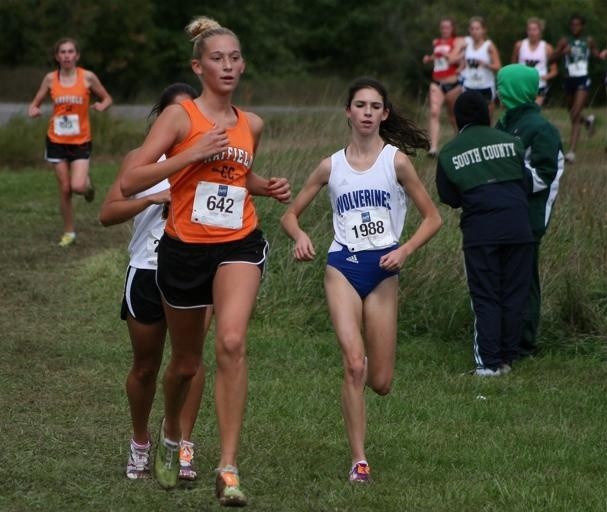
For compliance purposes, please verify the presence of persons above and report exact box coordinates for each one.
[27,38,113,250]
[119,15,292,508]
[435,89,542,376]
[419,14,607,163]
[281,78,443,488]
[492,63,565,356]
[96,81,215,482]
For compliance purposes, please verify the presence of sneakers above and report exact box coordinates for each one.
[216,465,247,505]
[473,368,501,376]
[179,440,197,481]
[127,432,151,479]
[586,114,595,138]
[59,232,76,248]
[86,173,94,202]
[563,151,576,162]
[155,416,179,488]
[349,461,369,484]
